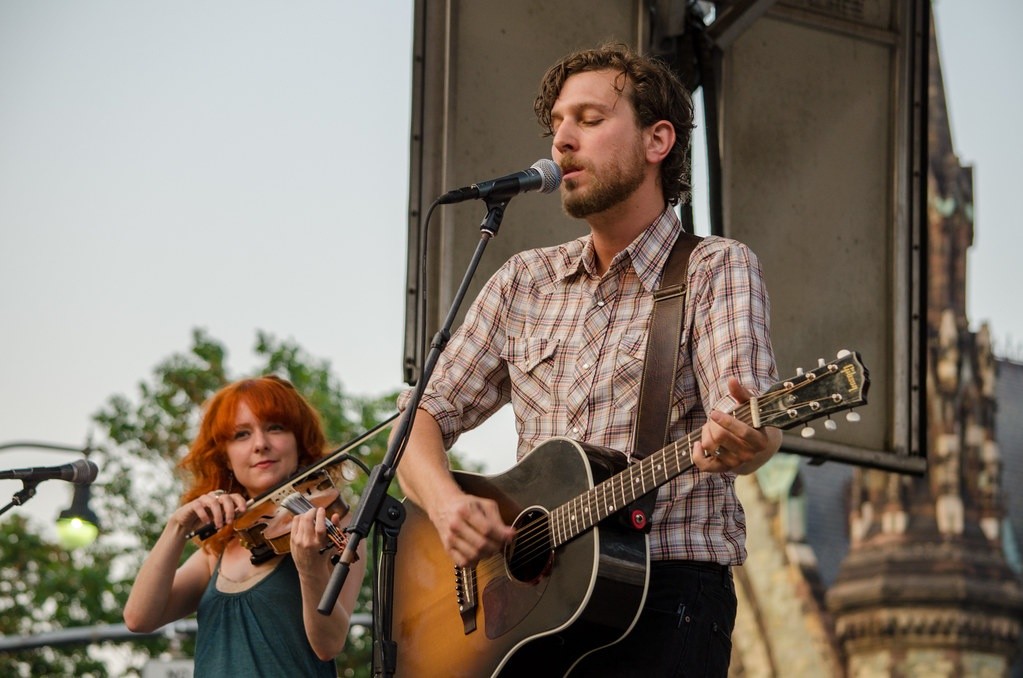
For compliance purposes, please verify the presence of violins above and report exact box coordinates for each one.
[233,474,360,565]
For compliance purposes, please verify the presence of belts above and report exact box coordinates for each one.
[650,561,734,578]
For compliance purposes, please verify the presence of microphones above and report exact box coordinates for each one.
[437,158,562,204]
[11,458,98,483]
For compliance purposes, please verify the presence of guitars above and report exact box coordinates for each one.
[390,348,872,677]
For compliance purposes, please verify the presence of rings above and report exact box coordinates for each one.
[711,445,724,458]
[213,490,224,501]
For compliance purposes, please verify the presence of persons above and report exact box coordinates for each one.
[123,375,367,678]
[386,42,783,678]
